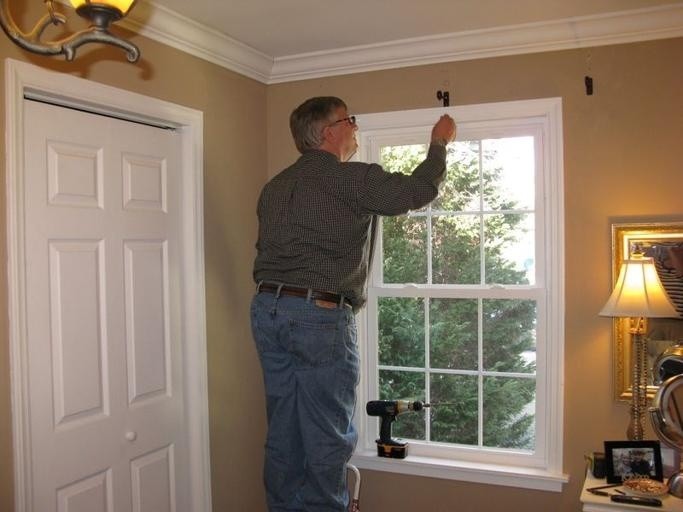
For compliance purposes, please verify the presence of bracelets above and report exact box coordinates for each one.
[429,139,447,147]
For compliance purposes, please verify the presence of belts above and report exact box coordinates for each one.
[259,283,353,306]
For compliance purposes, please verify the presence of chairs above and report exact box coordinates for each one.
[346,462,362,512]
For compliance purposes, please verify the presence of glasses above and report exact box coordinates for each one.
[335,115,358,123]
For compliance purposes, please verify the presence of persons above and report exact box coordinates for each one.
[629,449,651,475]
[616,449,632,474]
[246,95,458,512]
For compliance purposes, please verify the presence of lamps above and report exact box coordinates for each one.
[0,0,140,65]
[597,241,682,442]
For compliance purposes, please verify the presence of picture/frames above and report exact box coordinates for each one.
[603,439,664,484]
[610,222,683,407]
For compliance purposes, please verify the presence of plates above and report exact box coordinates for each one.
[623,476,672,498]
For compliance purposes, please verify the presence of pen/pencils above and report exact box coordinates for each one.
[587,484,625,496]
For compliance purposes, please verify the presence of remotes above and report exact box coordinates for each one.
[611,495,662,506]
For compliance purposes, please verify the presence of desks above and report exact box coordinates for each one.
[579,467,683,512]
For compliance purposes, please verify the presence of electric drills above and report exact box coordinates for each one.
[366,400,456,459]
